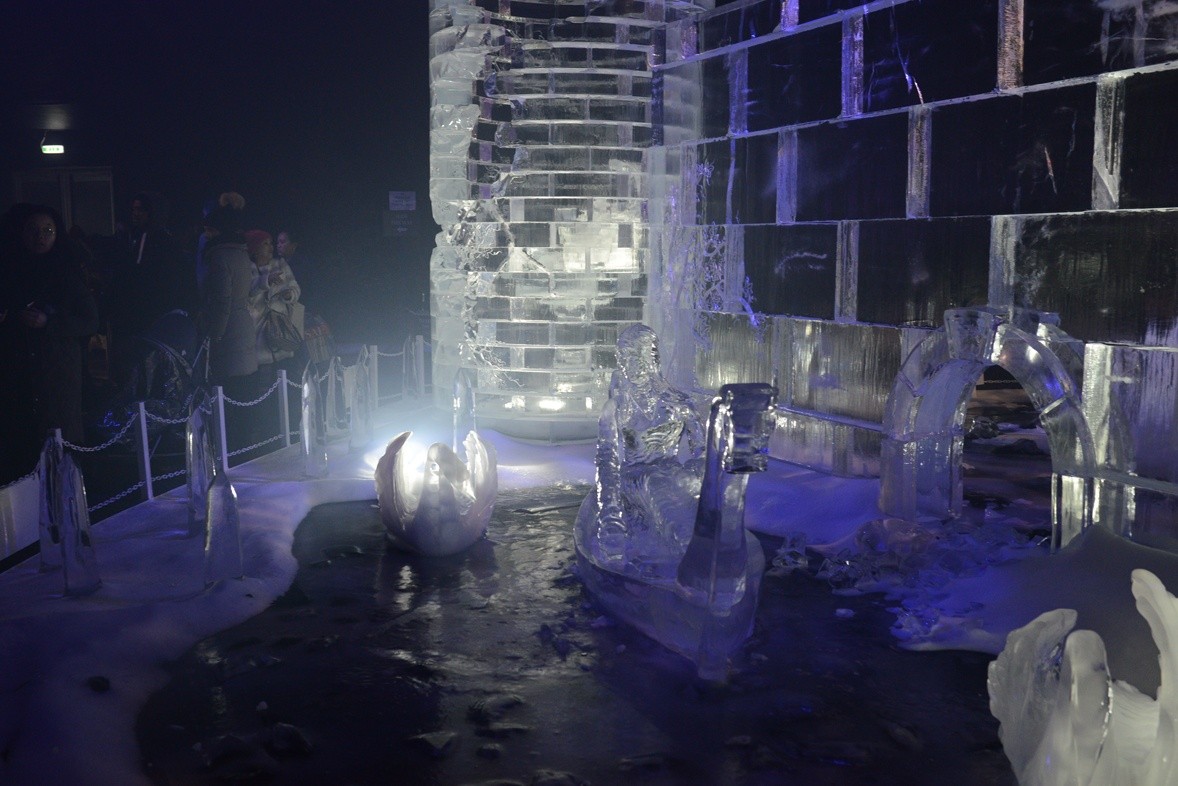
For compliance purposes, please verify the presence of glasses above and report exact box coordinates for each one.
[24,225,58,236]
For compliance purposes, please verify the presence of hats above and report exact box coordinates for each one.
[245,229,271,256]
[208,204,242,233]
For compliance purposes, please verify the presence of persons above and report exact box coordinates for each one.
[0,194,174,486]
[194,192,312,451]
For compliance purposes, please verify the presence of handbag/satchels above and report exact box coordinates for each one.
[263,309,301,350]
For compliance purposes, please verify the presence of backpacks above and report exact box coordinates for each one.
[133,332,219,440]
